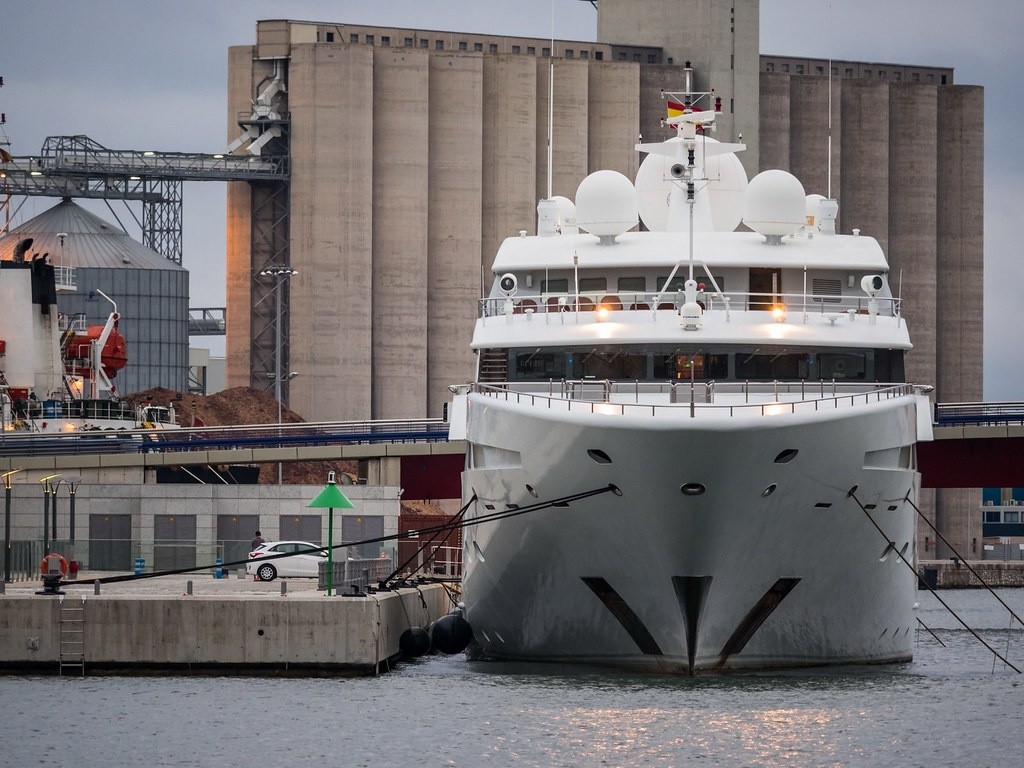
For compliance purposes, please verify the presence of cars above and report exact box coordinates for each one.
[245,540,335,582]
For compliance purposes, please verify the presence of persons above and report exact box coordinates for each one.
[251,531,265,552]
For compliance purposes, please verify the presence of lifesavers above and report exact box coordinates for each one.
[40,554,68,578]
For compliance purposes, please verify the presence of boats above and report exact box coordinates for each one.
[446,57,934,677]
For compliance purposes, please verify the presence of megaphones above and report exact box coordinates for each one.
[671,164,685,178]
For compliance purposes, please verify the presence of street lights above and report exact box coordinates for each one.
[40,473,65,580]
[48,475,64,553]
[260,261,301,485]
[64,476,83,580]
[1,467,29,581]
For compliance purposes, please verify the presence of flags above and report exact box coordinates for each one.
[667,102,708,132]
